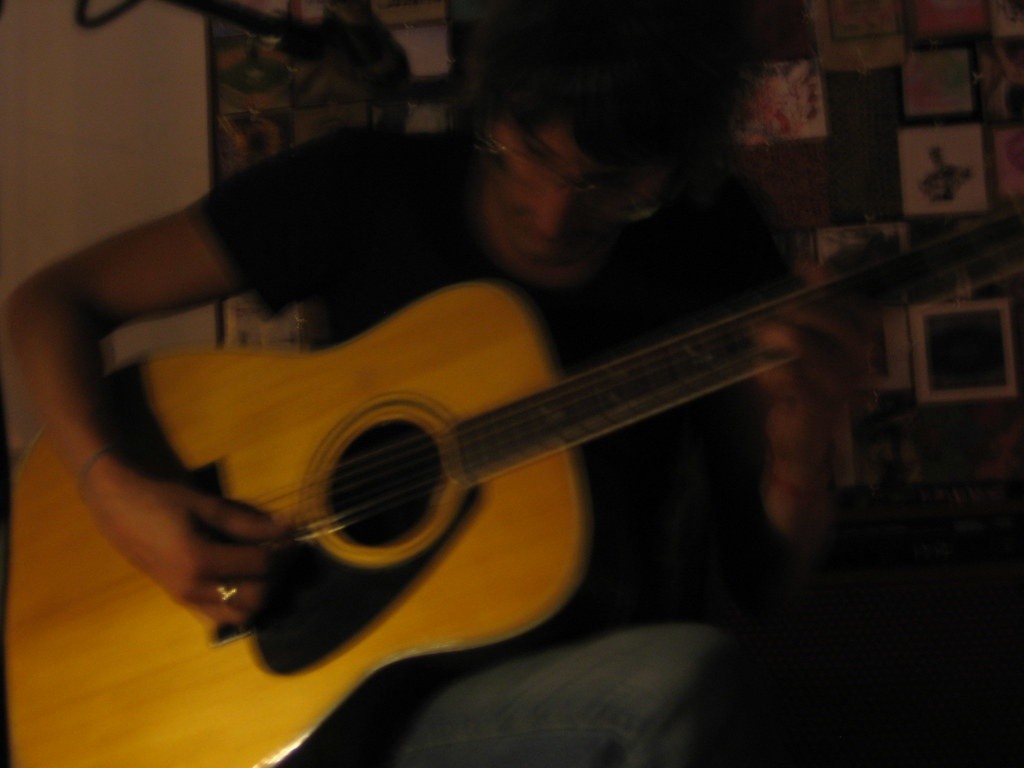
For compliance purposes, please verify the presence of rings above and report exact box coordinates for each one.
[218,578,240,601]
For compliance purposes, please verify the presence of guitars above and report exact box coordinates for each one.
[0,203,1022,766]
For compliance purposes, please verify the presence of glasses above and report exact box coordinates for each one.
[473,96,670,221]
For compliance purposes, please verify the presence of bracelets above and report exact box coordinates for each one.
[75,441,127,493]
[767,464,838,499]
[0,17,880,768]
